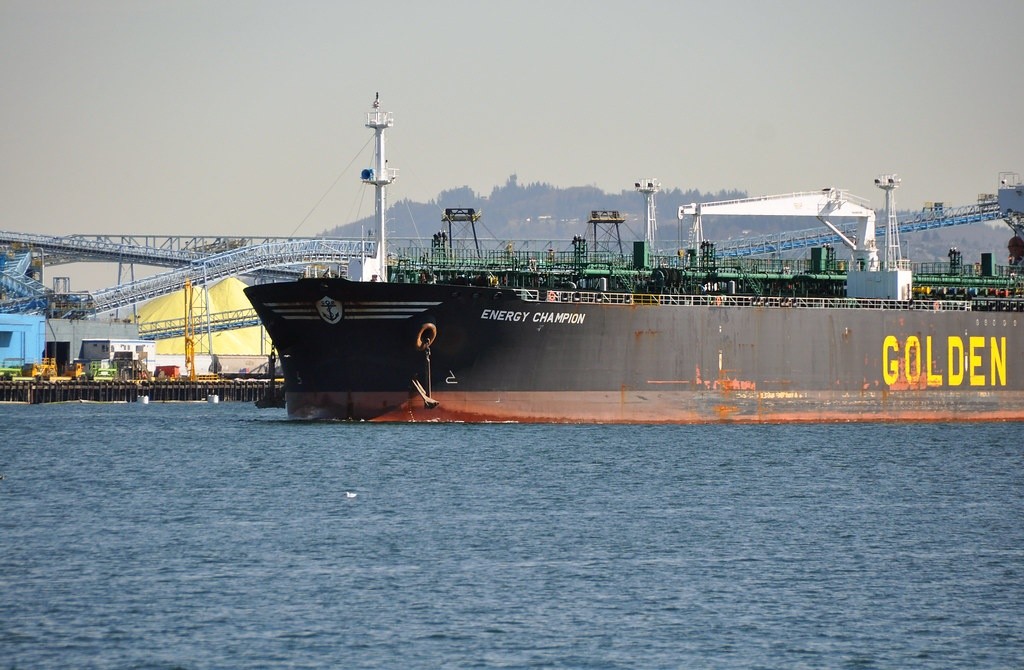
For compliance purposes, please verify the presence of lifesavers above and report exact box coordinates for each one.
[716,298,721,306]
[550,292,555,301]
[933,302,939,310]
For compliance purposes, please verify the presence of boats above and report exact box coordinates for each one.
[238,91,1024,427]
